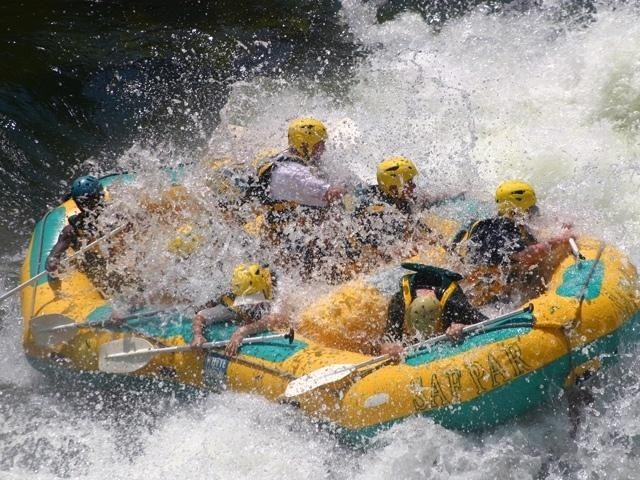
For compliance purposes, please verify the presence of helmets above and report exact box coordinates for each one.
[287,117,328,159]
[71,175,105,212]
[166,221,205,257]
[407,294,443,337]
[375,156,419,198]
[230,260,273,300]
[495,180,536,218]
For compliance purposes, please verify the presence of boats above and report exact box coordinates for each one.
[20,157,640,455]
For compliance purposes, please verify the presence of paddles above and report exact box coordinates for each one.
[285,303,534,398]
[30,305,182,345]
[98,328,295,373]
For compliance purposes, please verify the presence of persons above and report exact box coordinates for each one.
[380,263,489,362]
[333,157,466,278]
[45,176,143,277]
[240,118,348,245]
[190,262,290,356]
[450,181,578,305]
